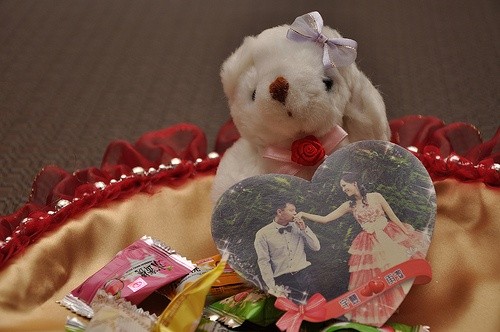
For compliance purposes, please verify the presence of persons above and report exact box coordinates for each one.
[293,173,431,329]
[254,199,345,320]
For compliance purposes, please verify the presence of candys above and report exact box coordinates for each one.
[55,234,432,332]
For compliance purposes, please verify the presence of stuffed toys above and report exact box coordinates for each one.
[212,10,391,202]
